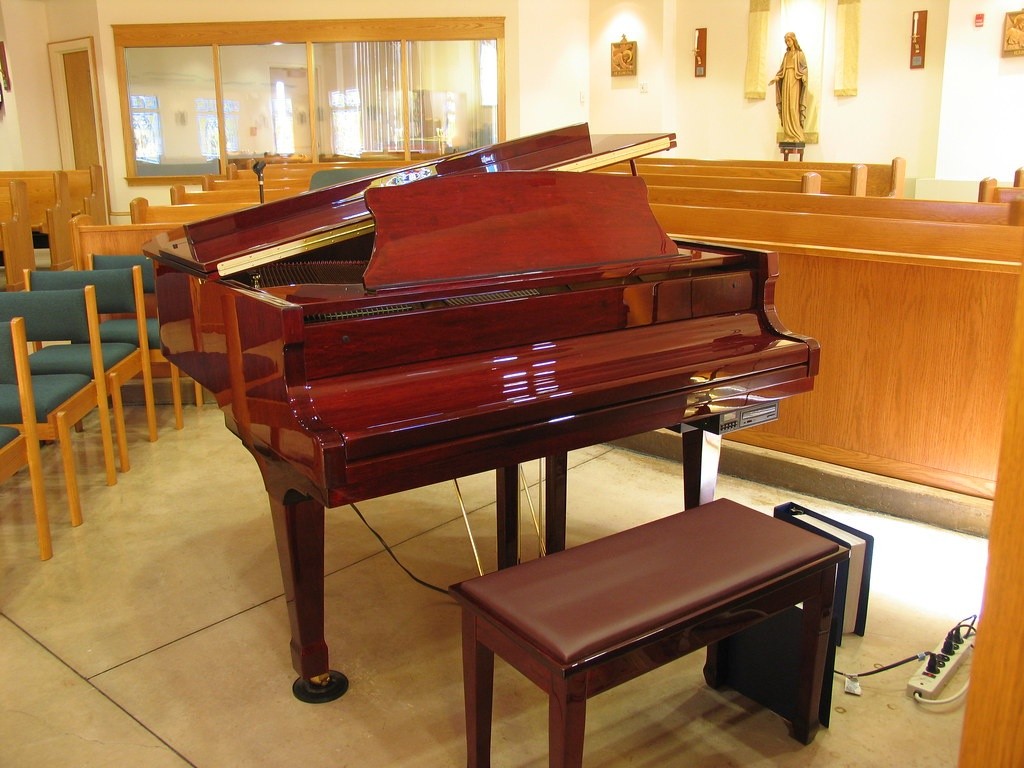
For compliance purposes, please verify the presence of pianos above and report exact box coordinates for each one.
[138,121,822,705]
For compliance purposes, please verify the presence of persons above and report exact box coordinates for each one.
[768,32,808,142]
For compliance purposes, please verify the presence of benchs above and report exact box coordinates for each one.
[449,498,852,768]
[0,165,108,293]
[69,156,1024,500]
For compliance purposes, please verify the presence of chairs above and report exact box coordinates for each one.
[0,252,205,560]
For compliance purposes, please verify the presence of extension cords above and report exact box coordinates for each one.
[906,636,972,700]
